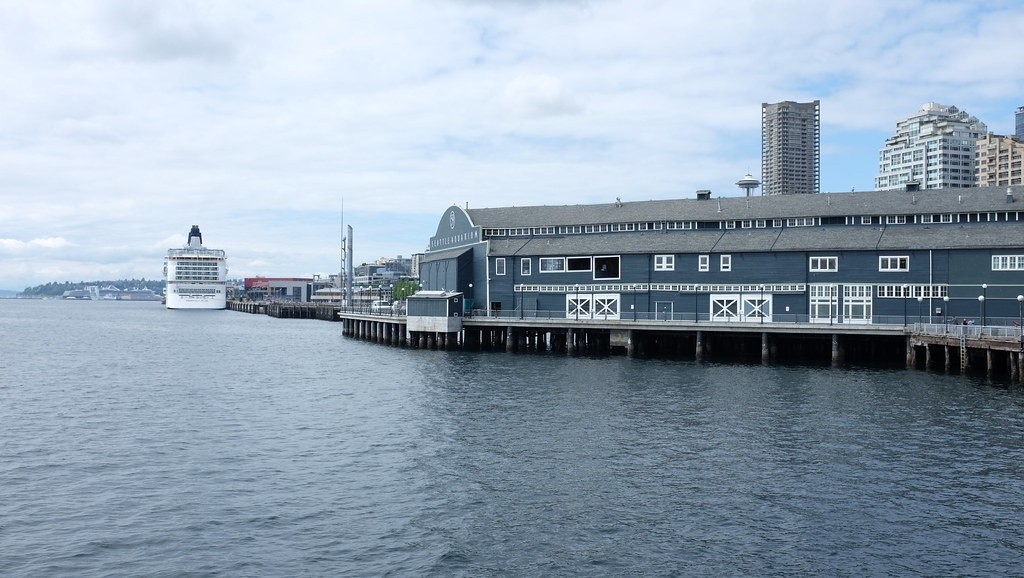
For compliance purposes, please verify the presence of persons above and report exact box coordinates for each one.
[951,315,958,325]
[963,318,975,326]
[1012,320,1021,336]
[595,264,611,278]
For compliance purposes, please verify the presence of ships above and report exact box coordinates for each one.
[163,224,230,310]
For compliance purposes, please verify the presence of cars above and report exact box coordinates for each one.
[399,306,406,315]
[391,300,405,316]
[359,286,364,315]
[371,300,392,312]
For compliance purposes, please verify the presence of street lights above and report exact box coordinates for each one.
[760,283,764,325]
[633,284,637,322]
[401,287,405,301]
[978,295,985,332]
[982,284,988,327]
[917,297,923,331]
[379,284,382,316]
[469,283,474,319]
[943,295,950,333]
[351,287,355,315]
[903,283,908,326]
[390,284,393,317]
[1017,295,1024,336]
[576,284,579,321]
[694,284,699,323]
[368,285,373,315]
[520,284,525,321]
